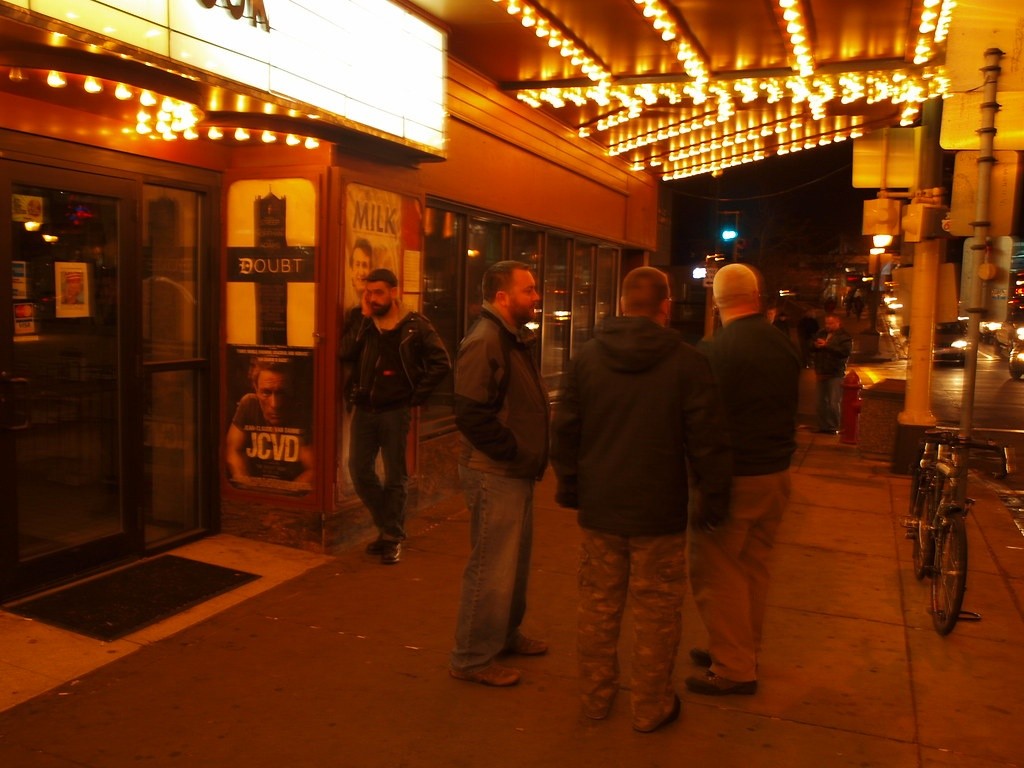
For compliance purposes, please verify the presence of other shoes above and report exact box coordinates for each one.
[817,426,836,435]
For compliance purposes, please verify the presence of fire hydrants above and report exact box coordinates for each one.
[837,368,864,446]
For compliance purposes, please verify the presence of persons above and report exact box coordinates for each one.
[688,262,796,694]
[346,237,372,329]
[807,314,851,433]
[552,267,732,732]
[340,270,450,560]
[824,297,835,314]
[766,299,819,368]
[453,260,551,685]
[843,294,864,320]
[226,359,313,494]
[61,271,84,304]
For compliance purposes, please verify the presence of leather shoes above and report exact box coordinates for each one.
[380,540,401,563]
[367,539,382,554]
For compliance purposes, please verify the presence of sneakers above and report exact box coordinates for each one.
[583,700,610,719]
[498,632,548,654]
[632,695,680,731]
[450,660,520,686]
[690,646,712,668]
[683,671,757,695]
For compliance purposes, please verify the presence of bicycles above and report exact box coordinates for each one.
[898,427,1008,637]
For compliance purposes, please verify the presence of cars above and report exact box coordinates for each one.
[979,320,1024,379]
[933,320,966,366]
